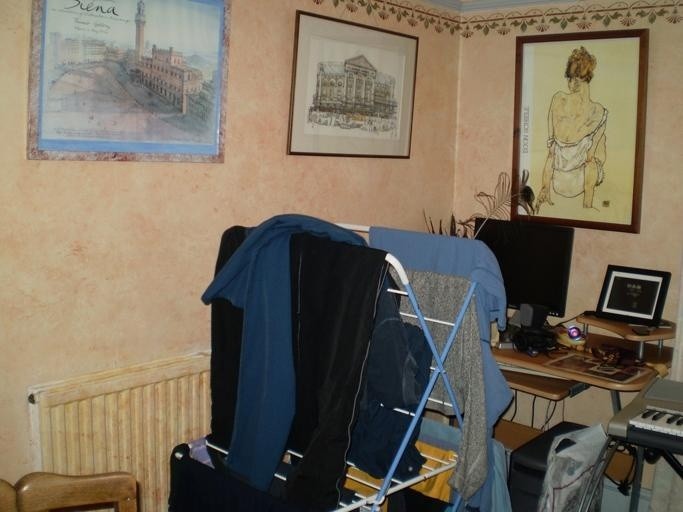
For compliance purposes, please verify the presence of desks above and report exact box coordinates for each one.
[483,312,675,498]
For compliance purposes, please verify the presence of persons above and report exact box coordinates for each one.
[534,46,608,214]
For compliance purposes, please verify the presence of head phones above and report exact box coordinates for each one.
[514,329,555,353]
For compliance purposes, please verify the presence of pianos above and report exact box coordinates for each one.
[608,378,683,456]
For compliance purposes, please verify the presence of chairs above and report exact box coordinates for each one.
[0,467,137,512]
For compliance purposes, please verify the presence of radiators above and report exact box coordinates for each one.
[27,349,213,511]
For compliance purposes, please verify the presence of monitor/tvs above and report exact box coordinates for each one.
[474,217,574,329]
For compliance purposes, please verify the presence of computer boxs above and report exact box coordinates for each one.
[507,422,590,512]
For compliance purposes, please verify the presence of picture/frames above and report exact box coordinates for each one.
[509,28,649,234]
[596,262,672,326]
[27,0,232,164]
[286,9,419,160]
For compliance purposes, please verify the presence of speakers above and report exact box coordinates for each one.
[519,303,549,330]
